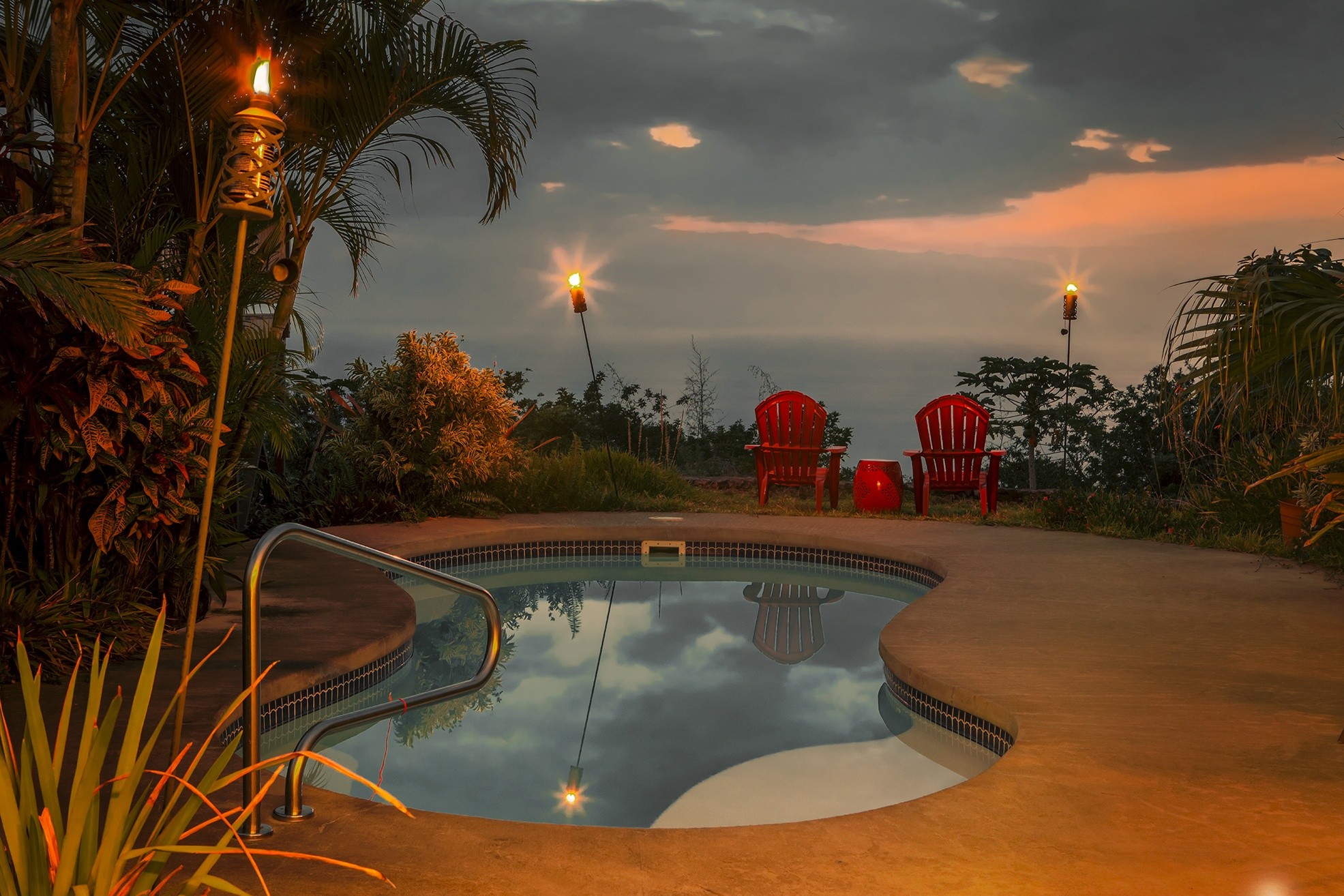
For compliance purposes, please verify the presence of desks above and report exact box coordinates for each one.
[853,458,904,514]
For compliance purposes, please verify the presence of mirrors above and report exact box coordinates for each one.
[742,582,848,668]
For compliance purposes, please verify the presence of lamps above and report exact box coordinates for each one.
[567,271,589,315]
[1062,283,1079,320]
[214,58,289,219]
[565,764,584,804]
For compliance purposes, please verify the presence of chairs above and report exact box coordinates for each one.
[744,390,849,514]
[902,393,1008,517]
